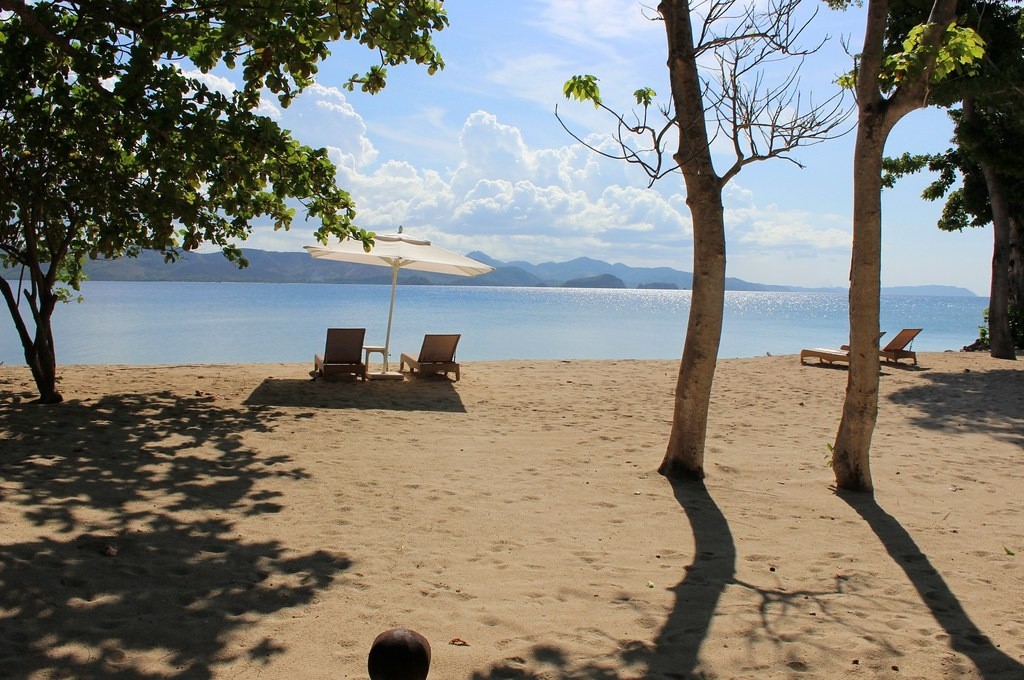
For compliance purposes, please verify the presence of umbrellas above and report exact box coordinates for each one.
[300,225,498,375]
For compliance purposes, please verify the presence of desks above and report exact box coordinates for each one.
[363,346,385,372]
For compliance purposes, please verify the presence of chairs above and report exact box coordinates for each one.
[801,331,886,368]
[314,327,366,383]
[399,334,461,382]
[879,328,923,365]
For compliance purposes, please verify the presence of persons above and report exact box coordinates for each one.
[965,328,990,352]
